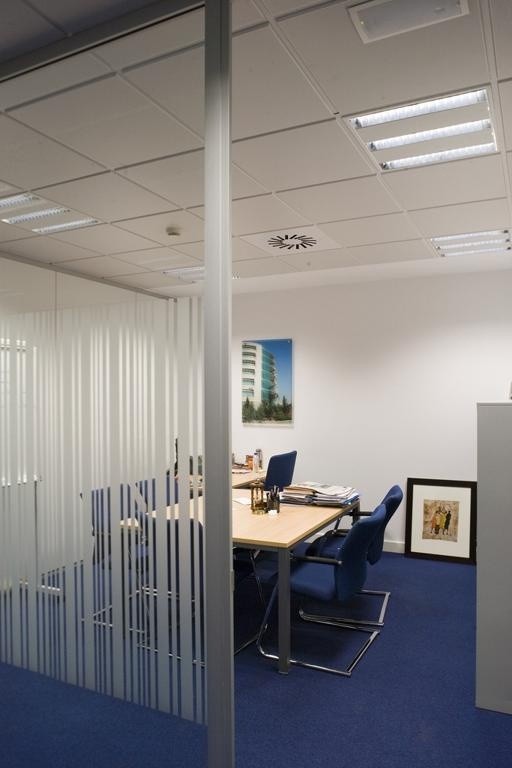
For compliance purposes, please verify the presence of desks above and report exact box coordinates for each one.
[174,468,268,492]
[121,488,360,675]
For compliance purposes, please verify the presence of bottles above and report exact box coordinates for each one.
[246,449,263,473]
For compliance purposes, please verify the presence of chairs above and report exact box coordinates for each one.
[263,450,297,493]
[135,511,268,668]
[135,475,178,529]
[304,485,404,628]
[80,482,192,636]
[255,504,387,678]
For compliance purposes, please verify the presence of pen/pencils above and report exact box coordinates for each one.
[267,485,280,500]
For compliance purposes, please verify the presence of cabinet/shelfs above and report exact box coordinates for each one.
[476,402,512,715]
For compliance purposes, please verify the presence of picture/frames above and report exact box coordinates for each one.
[404,477,477,566]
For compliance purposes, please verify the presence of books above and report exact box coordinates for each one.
[280,477,360,510]
[429,505,452,535]
